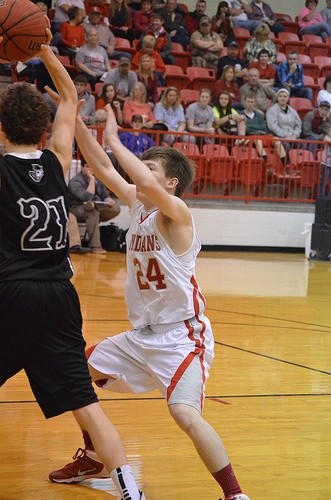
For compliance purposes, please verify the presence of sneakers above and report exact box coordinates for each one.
[219,493,249,500]
[49,448,111,484]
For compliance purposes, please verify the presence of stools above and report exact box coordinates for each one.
[77,222,89,240]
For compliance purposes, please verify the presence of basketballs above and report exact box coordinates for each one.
[0,0,50,65]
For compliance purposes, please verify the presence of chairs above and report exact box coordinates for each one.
[1,1,331,200]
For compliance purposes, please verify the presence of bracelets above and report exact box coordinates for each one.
[227,114,233,119]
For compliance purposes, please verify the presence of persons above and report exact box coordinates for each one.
[186,0,331,149]
[67,159,121,253]
[239,93,291,165]
[119,115,155,161]
[186,88,217,155]
[16,0,191,110]
[301,101,331,157]
[97,82,125,127]
[0,15,146,500]
[213,92,249,157]
[44,85,249,500]
[267,89,317,161]
[153,88,196,149]
[67,212,91,254]
[74,76,96,126]
[123,82,168,145]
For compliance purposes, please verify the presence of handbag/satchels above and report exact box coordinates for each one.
[99,225,125,251]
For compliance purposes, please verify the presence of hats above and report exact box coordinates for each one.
[200,16,211,26]
[229,40,238,47]
[119,57,130,66]
[277,88,290,97]
[319,101,330,109]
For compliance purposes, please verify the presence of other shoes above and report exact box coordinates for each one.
[83,202,95,211]
[70,245,91,254]
[88,246,107,254]
